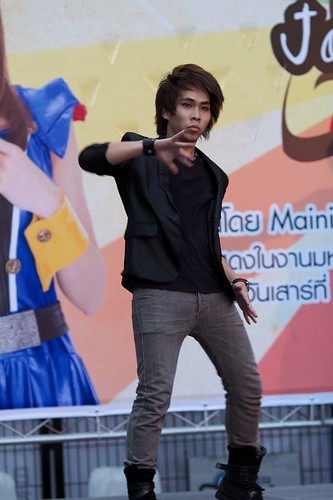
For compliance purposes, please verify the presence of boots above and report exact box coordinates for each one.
[123,467,157,500]
[214,439,266,500]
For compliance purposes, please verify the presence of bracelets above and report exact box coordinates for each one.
[140,137,156,157]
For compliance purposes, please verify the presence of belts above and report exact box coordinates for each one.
[0,301,69,354]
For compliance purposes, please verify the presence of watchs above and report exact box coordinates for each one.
[230,277,249,292]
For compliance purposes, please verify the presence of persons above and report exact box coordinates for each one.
[72,62,269,500]
[0,10,106,408]
